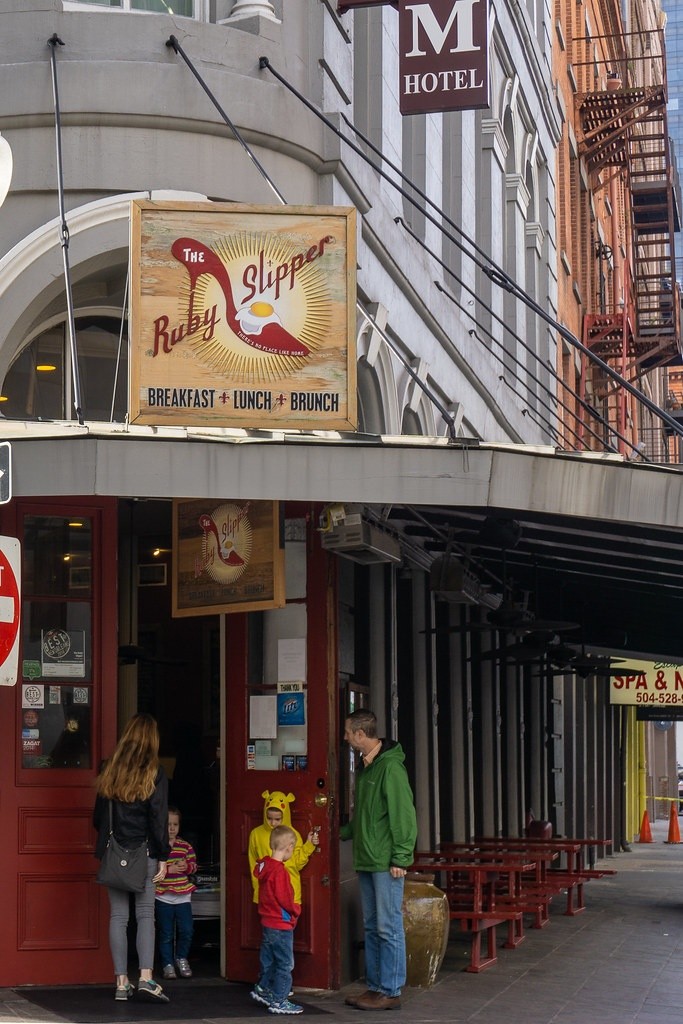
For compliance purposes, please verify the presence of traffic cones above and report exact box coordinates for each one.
[664,801,683,845]
[635,811,657,843]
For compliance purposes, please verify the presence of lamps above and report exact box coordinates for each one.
[317,501,348,531]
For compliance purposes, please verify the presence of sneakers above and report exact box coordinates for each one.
[114,983,136,1001]
[161,962,176,978]
[136,977,170,1002]
[175,957,192,977]
[249,983,274,1007]
[267,997,304,1014]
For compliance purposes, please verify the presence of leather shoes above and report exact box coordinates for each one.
[345,989,376,1005]
[355,993,401,1011]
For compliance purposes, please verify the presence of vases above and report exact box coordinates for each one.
[401,870,452,987]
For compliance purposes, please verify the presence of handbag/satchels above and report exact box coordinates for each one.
[95,833,150,893]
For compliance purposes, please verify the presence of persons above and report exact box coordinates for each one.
[249,825,304,1015]
[92,712,173,1005]
[155,804,200,979]
[248,789,320,997]
[337,707,420,1010]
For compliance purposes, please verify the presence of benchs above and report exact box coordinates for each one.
[408,833,621,973]
